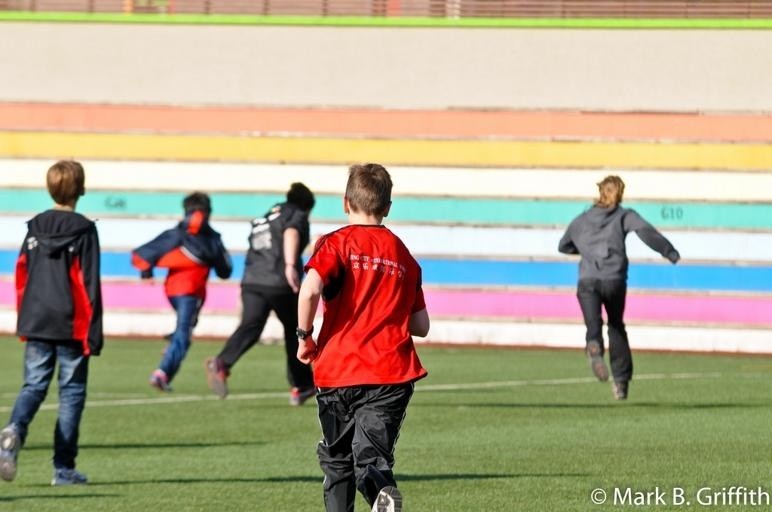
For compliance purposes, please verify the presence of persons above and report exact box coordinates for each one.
[297,164,429,512]
[0,161,104,486]
[132,193,231,391]
[559,175,680,399]
[206,183,319,406]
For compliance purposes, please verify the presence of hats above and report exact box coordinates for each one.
[288,181,316,210]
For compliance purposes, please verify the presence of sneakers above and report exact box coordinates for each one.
[50,468,89,486]
[369,486,404,512]
[205,355,229,400]
[149,369,174,395]
[289,386,318,408]
[587,338,608,384]
[610,380,629,400]
[0,421,26,483]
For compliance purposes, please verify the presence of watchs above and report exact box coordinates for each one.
[296,326,314,339]
